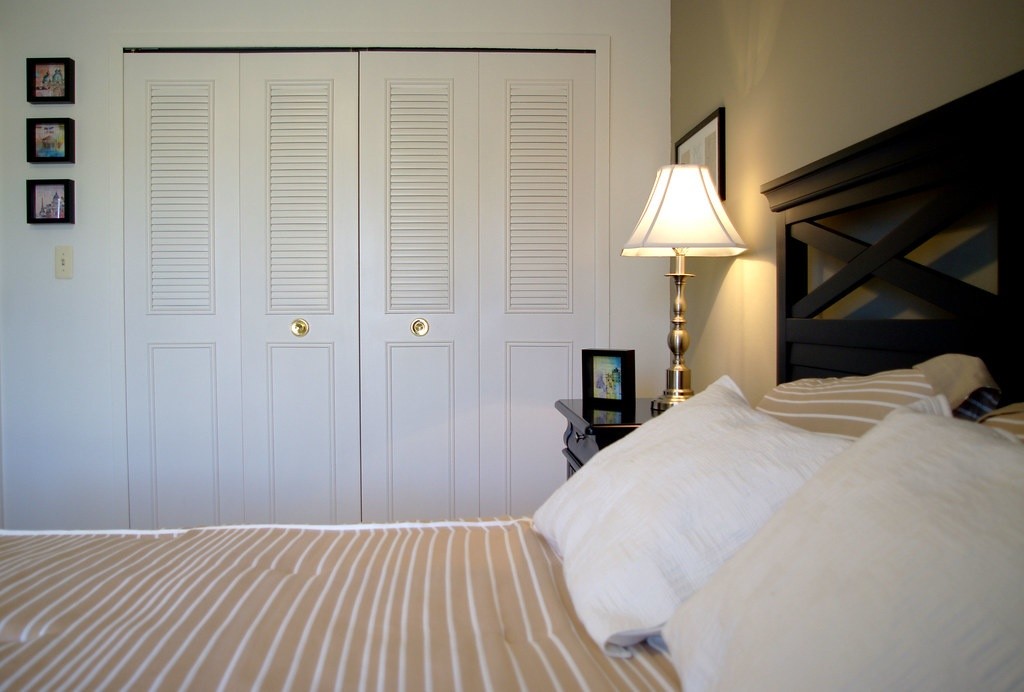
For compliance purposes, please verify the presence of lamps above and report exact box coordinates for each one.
[620,161,748,413]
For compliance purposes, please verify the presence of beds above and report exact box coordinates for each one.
[0,67,1024,692]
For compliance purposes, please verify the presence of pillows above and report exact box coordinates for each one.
[647,395,1024,692]
[529,373,855,657]
[756,353,1003,438]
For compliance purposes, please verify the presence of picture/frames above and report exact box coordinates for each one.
[26,118,76,164]
[675,105,726,203]
[26,57,76,104]
[26,179,75,225]
[581,348,636,415]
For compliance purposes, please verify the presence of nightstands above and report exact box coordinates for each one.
[555,397,663,483]
